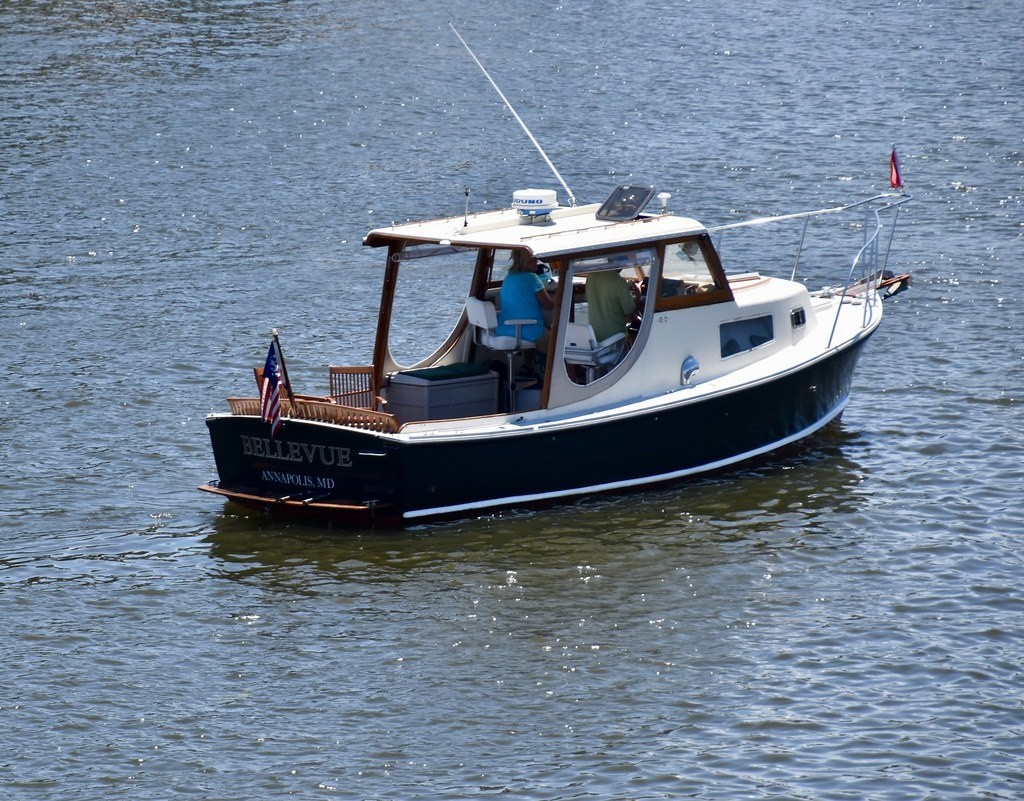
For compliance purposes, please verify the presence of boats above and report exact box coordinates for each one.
[195,31,927,537]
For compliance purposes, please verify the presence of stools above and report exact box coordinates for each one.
[386,370,500,426]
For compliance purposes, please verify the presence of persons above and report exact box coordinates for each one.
[587,269,637,342]
[495,248,555,354]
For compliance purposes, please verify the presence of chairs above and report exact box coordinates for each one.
[254,366,288,398]
[564,323,626,383]
[465,296,540,413]
[328,365,388,414]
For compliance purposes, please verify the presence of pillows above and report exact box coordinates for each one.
[399,363,490,381]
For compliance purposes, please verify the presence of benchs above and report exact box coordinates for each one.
[227,396,398,434]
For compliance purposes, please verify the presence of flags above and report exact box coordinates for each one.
[890,150,902,188]
